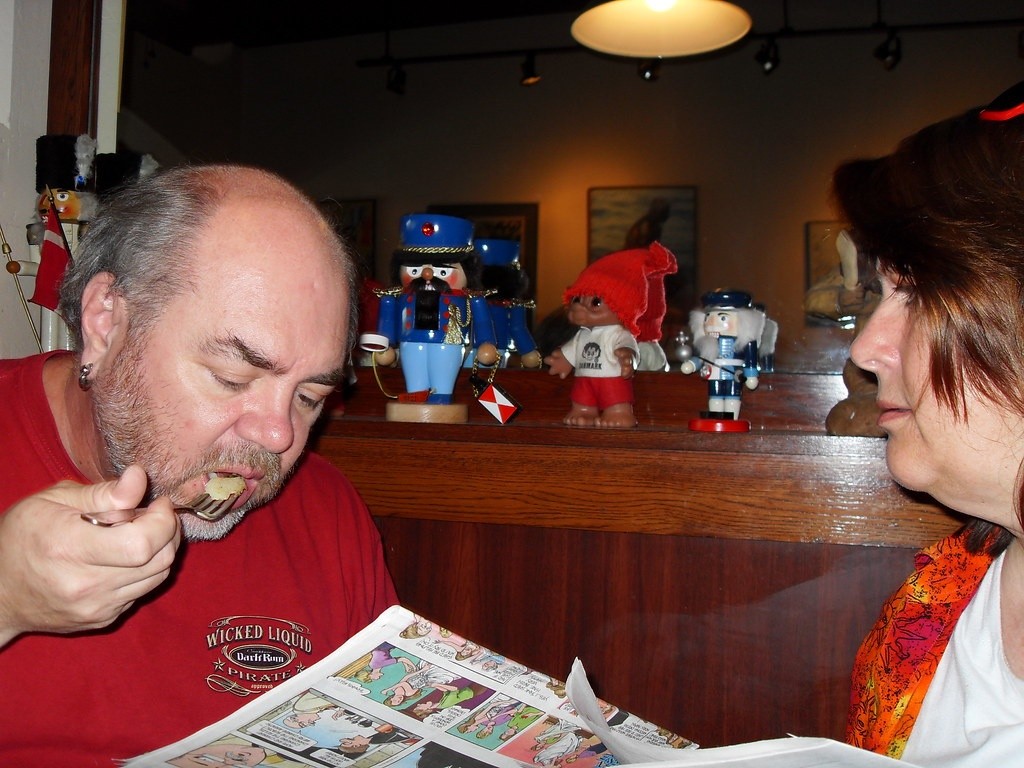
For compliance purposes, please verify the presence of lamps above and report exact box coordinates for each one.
[518,53,541,87]
[867,28,905,67]
[572,0,755,59]
[640,61,659,81]
[756,39,780,74]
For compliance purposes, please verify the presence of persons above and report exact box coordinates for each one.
[0,166,399,768]
[834,82,1024,767]
[680,289,765,420]
[754,303,778,373]
[462,240,543,370]
[321,195,498,419]
[6,133,91,355]
[637,249,676,371]
[544,242,667,426]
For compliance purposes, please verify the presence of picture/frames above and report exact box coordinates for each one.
[585,184,699,311]
[807,222,892,330]
[427,203,538,332]
[320,199,375,287]
